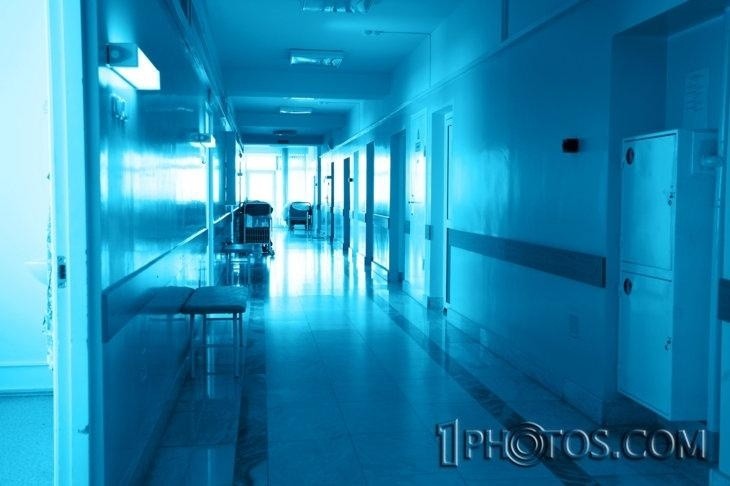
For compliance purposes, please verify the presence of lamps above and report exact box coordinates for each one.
[108,42,164,96]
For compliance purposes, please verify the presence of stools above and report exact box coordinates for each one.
[184,285,248,379]
[223,242,255,281]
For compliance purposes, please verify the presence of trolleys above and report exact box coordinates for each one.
[288,201,311,231]
[238,202,274,256]
